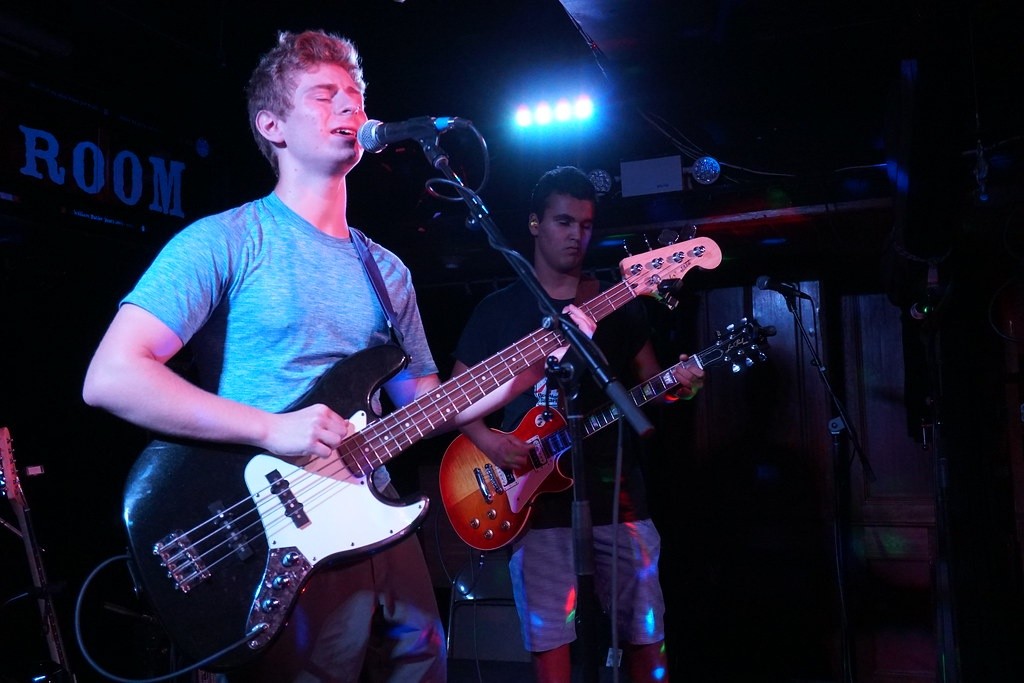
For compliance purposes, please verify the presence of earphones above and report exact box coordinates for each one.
[531,221,538,226]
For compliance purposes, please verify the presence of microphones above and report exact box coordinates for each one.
[756,275,812,299]
[357,116,471,154]
[910,287,948,320]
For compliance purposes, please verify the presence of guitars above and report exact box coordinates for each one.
[120,222,727,671]
[0,424,73,682]
[436,315,771,557]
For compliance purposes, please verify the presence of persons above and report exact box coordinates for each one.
[447,164,707,683]
[81,31,595,683]
